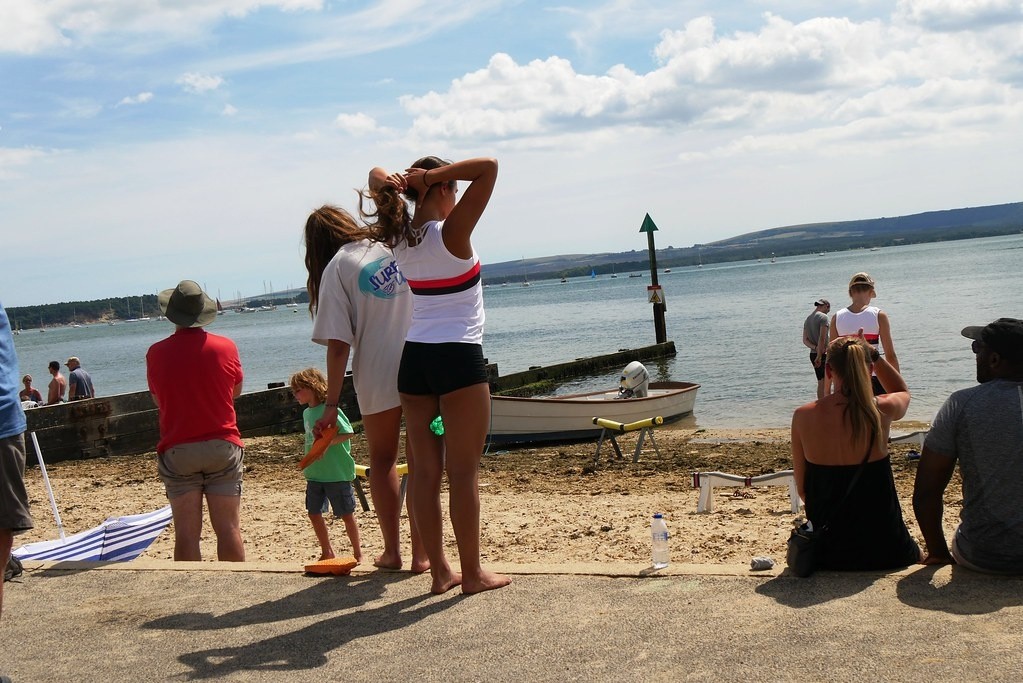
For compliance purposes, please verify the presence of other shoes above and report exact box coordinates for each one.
[2,555,23,581]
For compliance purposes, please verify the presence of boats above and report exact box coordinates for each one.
[629,272,643,278]
[561,276,569,283]
[664,267,671,272]
[696,264,702,268]
[485,361,700,445]
[501,282,507,287]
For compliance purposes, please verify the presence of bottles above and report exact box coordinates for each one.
[59,399,64,404]
[430,416,444,435]
[28,403,38,408]
[651,514,669,569]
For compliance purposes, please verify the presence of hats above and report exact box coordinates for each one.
[64,357,79,366]
[159,281,219,326]
[814,299,830,312]
[849,272,877,298]
[960,318,1023,363]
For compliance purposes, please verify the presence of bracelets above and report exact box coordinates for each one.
[870,349,879,362]
[816,356,822,359]
[815,345,817,351]
[325,404,338,407]
[423,170,430,187]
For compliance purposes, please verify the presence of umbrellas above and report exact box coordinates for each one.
[10,432,173,561]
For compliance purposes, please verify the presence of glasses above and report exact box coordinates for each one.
[971,340,996,354]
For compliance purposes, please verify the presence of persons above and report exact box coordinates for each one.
[292,370,364,562]
[912,316,1023,573]
[823,272,899,397]
[358,154,513,594]
[0,301,35,682]
[64,357,95,401]
[47,361,66,404]
[19,374,43,402]
[145,279,249,562]
[792,328,924,574]
[802,298,833,400]
[302,205,430,573]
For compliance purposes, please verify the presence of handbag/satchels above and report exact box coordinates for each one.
[785,524,819,578]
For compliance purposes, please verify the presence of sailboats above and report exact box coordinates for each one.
[105,287,168,326]
[591,269,595,278]
[521,255,529,286]
[71,307,81,328]
[216,279,299,315]
[39,317,45,333]
[611,264,618,278]
